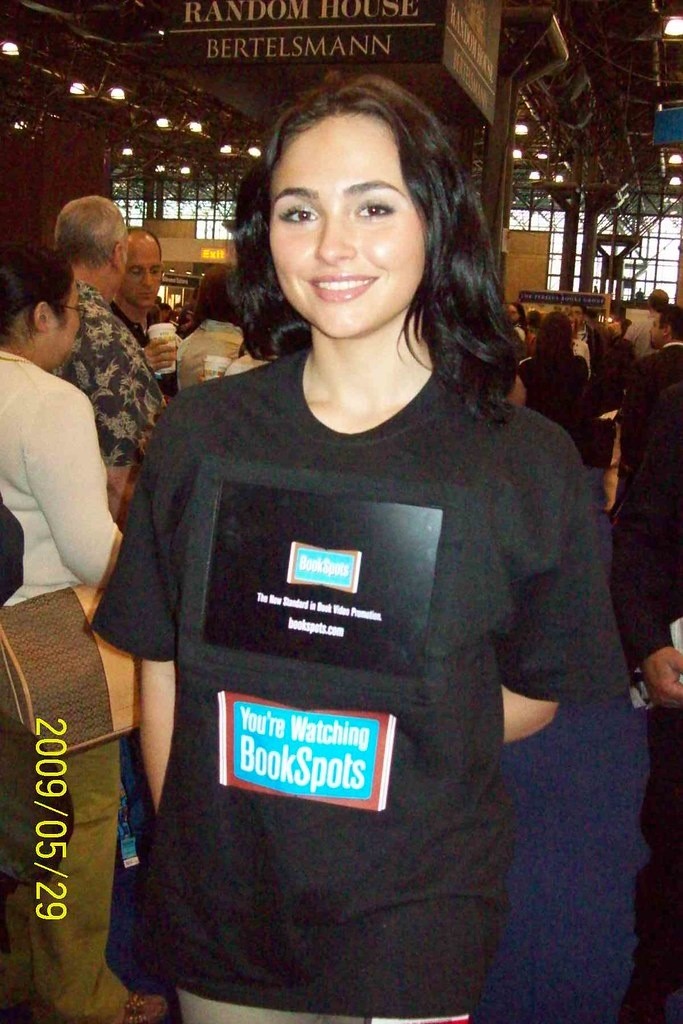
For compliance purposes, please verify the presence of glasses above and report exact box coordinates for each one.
[49,302,88,319]
[125,264,166,280]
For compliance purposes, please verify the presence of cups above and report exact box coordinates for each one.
[147,322,177,374]
[202,355,232,381]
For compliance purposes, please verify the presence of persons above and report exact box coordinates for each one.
[607,383,683,1023]
[90,72,597,1024]
[0,195,178,1024]
[507,289,683,526]
[175,263,272,391]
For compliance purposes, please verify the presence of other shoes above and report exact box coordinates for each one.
[123,992,166,1024]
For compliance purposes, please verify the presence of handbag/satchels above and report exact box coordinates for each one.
[0,586,148,758]
